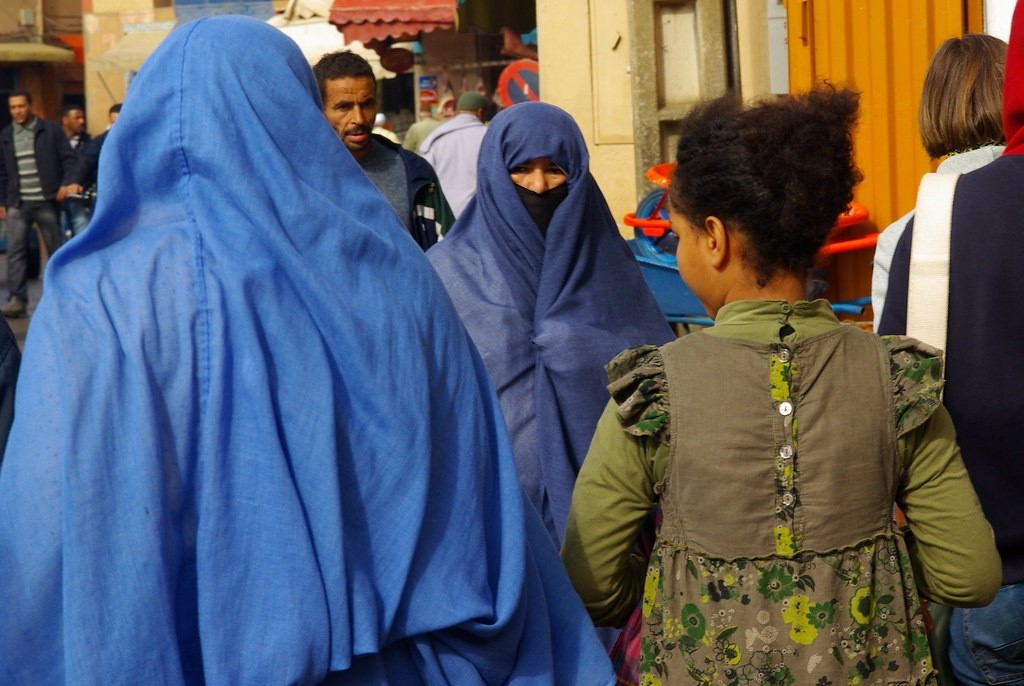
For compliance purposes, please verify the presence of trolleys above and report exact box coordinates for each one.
[623,238,873,336]
[623,160,880,267]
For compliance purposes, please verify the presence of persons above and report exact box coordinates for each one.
[561,86,1002,686]
[0,91,124,319]
[0,16,619,686]
[312,50,457,251]
[372,100,439,155]
[870,0,1024,686]
[419,92,495,222]
[424,101,679,551]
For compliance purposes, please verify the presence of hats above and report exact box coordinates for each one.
[457,92,491,110]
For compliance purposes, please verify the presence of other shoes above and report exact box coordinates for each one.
[1,296,26,317]
